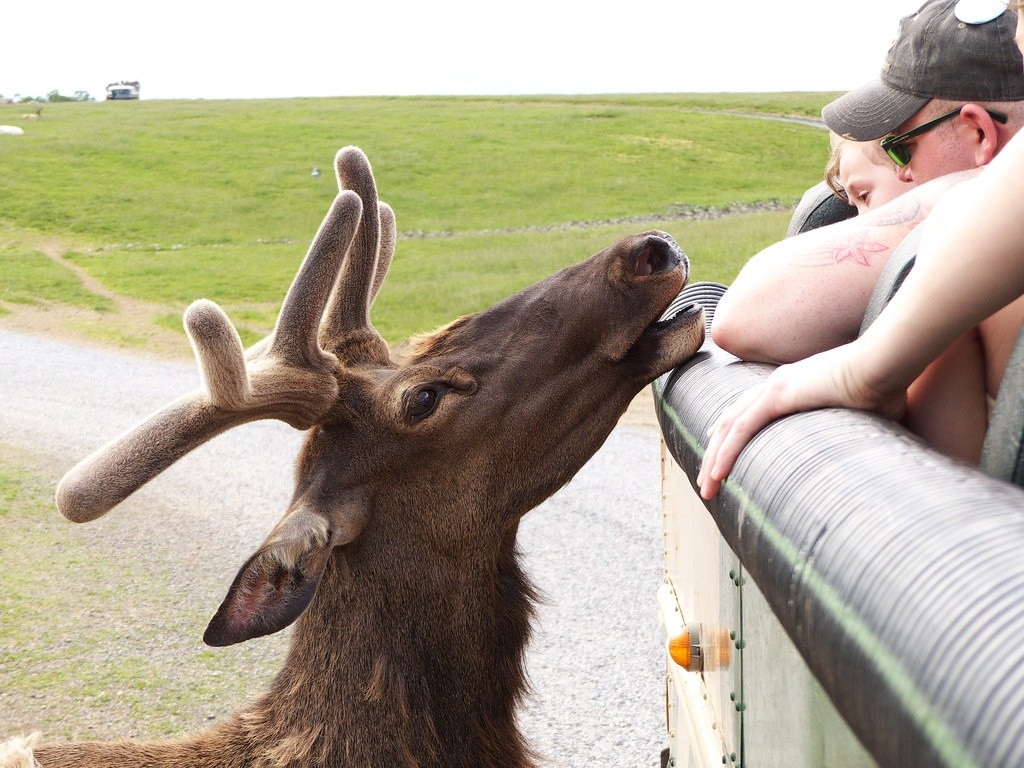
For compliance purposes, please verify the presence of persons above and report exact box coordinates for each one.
[698,0,1024,505]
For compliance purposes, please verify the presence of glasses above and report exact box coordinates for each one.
[880,107,1008,167]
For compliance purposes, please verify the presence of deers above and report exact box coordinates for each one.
[0,146,706,767]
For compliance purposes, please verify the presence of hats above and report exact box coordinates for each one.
[823,0,1024,142]
